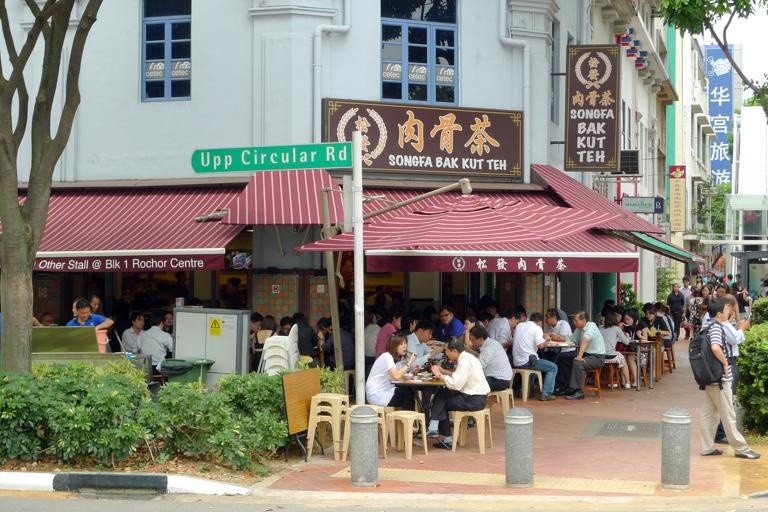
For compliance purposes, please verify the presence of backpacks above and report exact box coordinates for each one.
[688,322,727,391]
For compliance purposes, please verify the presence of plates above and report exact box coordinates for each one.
[420,378,432,381]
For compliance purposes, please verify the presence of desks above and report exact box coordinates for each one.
[283,368,326,463]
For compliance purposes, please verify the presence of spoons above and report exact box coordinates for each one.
[623,322,629,327]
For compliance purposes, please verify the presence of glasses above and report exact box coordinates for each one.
[440,312,450,318]
[390,332,400,340]
[573,319,578,325]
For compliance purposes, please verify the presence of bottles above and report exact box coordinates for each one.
[415,365,421,376]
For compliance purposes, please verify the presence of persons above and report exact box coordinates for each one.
[485,299,677,401]
[215,278,355,394]
[32,271,188,374]
[666,271,754,342]
[363,285,513,451]
[698,294,761,459]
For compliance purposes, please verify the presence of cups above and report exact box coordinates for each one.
[651,328,661,337]
[175,298,184,309]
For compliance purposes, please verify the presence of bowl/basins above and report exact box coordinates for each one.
[547,342,557,346]
[568,342,573,345]
[404,374,413,378]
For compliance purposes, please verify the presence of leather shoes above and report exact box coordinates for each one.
[416,427,439,439]
[565,391,584,400]
[433,440,458,448]
[559,389,576,396]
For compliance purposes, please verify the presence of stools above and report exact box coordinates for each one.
[257,324,299,377]
[508,368,542,401]
[582,368,601,399]
[618,334,676,392]
[306,392,428,463]
[487,389,514,420]
[152,374,166,385]
[451,408,493,455]
[598,363,621,391]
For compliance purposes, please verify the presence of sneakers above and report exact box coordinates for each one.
[607,384,616,388]
[735,450,759,459]
[623,384,629,389]
[701,450,723,456]
[540,392,555,401]
[714,438,727,443]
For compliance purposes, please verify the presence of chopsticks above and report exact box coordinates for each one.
[404,350,417,357]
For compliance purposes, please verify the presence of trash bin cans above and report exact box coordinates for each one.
[161,358,215,384]
